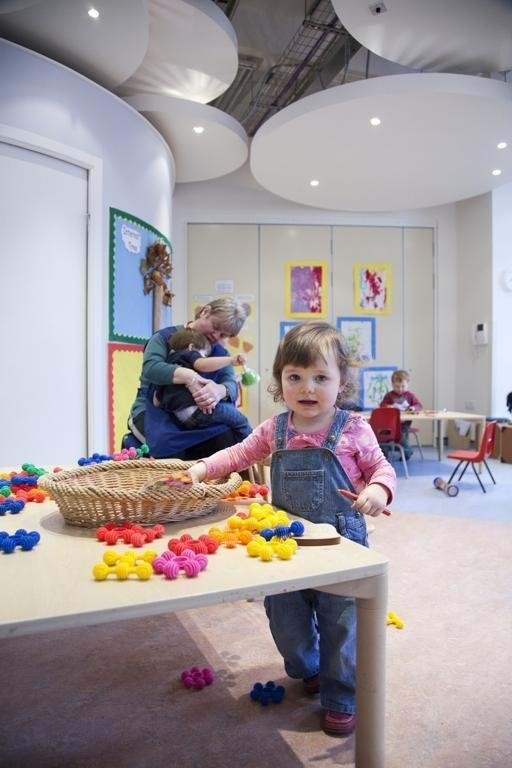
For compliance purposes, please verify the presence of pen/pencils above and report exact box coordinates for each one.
[337,489,392,516]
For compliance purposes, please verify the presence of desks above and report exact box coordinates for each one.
[359,412,486,475]
[0,496,389,768]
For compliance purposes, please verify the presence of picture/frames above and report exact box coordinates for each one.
[280,322,298,340]
[285,260,328,317]
[361,368,397,410]
[338,317,376,362]
[354,262,392,315]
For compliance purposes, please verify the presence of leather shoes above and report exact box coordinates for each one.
[302,673,319,694]
[323,708,357,736]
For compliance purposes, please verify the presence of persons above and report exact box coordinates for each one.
[122,298,259,484]
[165,330,255,438]
[381,371,422,460]
[158,321,395,733]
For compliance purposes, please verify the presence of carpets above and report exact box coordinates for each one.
[0,509,512,768]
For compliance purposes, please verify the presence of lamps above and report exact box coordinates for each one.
[370,4,386,17]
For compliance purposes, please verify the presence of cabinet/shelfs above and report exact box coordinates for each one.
[494,423,512,464]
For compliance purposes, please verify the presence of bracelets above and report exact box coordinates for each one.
[184,372,198,389]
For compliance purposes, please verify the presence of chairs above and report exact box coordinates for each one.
[447,420,496,493]
[369,407,409,480]
[408,427,423,461]
